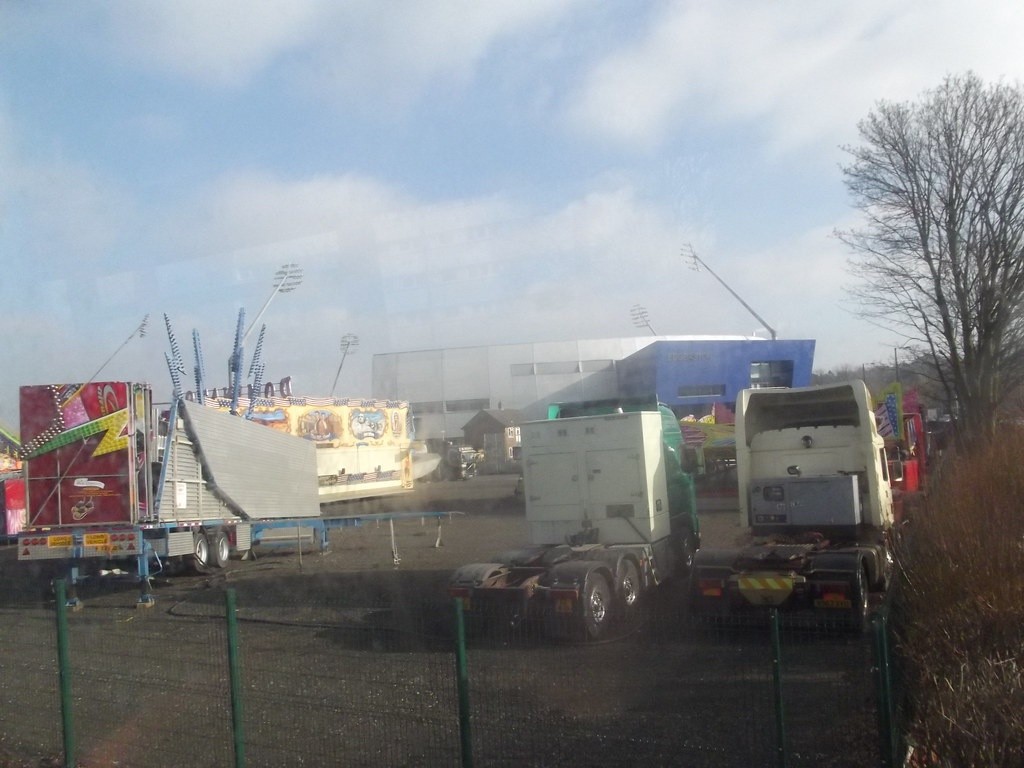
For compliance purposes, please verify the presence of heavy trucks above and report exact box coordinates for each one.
[444,395,701,640]
[690,380,900,640]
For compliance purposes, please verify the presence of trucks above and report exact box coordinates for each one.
[419,437,478,481]
